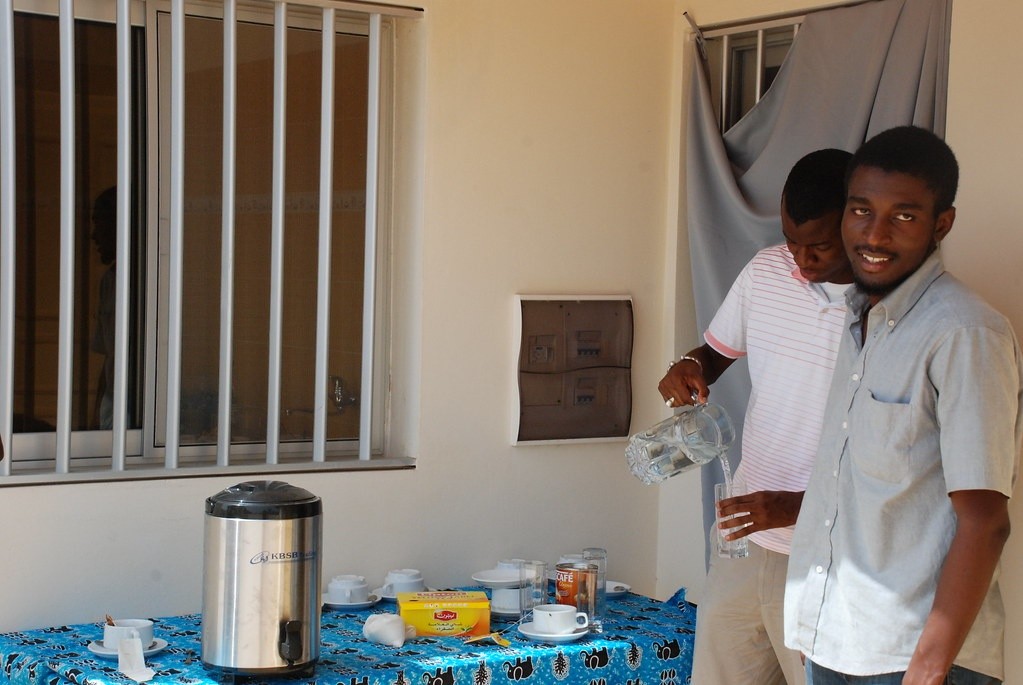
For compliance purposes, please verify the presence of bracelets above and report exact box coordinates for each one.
[667,355,702,371]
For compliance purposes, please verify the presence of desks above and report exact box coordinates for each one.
[0,579,697,685]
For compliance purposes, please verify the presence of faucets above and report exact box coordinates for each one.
[278,620,303,671]
[285,373,359,417]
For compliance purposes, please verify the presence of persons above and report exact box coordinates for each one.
[91,188,116,429]
[784,125,1023,685]
[656,147,854,685]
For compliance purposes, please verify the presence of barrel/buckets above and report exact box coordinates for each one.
[201,481,321,684]
[554,563,598,617]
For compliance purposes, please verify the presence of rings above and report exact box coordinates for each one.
[666,401,671,406]
[669,398,674,403]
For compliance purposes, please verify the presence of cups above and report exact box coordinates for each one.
[497,559,525,569]
[381,569,425,598]
[518,560,548,624]
[712,480,751,560]
[578,548,607,629]
[103,618,154,651]
[532,604,589,634]
[328,575,369,605]
[491,588,546,613]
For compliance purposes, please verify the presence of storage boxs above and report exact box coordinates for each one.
[397,591,490,636]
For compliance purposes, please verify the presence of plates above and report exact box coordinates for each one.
[547,569,558,585]
[596,580,632,596]
[517,622,590,643]
[489,609,528,618]
[372,587,440,602]
[88,637,168,660]
[321,593,381,608]
[473,568,537,589]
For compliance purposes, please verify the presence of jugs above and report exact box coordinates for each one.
[626,391,736,484]
